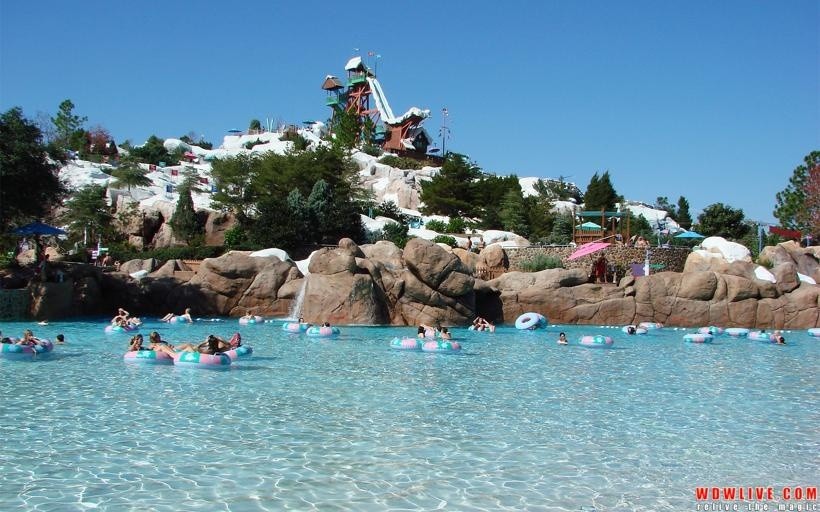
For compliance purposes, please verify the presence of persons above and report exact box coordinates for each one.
[627,325,638,334]
[478,236,485,255]
[774,330,785,344]
[298,318,308,325]
[0,304,242,361]
[596,252,608,284]
[635,236,646,247]
[557,332,568,345]
[614,230,623,253]
[465,235,472,253]
[243,309,254,319]
[417,316,496,341]
[321,320,330,327]
[14,237,122,285]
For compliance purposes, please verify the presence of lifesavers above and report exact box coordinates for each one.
[389,326,461,353]
[468,323,494,331]
[683,325,776,344]
[283,323,339,337]
[239,315,264,324]
[622,322,664,334]
[514,312,547,330]
[0,337,53,353]
[167,316,189,323]
[576,335,612,346]
[124,344,253,364]
[808,328,820,337]
[105,320,138,332]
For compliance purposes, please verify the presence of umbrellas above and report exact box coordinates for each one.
[575,221,607,231]
[565,242,611,263]
[673,230,705,247]
[7,221,68,241]
